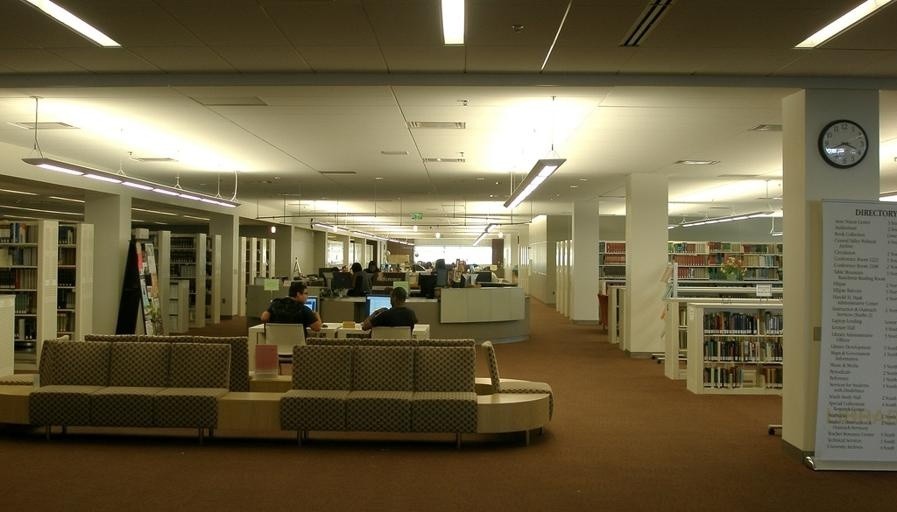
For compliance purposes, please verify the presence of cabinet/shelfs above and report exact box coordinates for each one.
[597,242,784,395]
[0,218,276,372]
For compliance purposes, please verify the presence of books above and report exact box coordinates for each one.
[0,216,268,340]
[598,242,782,387]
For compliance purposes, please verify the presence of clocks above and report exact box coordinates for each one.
[818,120,868,169]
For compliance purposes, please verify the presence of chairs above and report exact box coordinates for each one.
[0,334,71,425]
[27,322,554,446]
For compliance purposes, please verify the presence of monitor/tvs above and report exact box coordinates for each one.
[365,294,392,318]
[303,296,318,313]
[475,271,491,282]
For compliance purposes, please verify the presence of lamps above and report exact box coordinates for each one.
[471,218,498,247]
[503,145,566,211]
[21,95,242,207]
[668,179,784,237]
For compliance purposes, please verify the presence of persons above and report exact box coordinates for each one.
[258,282,321,359]
[351,262,372,289]
[361,287,417,340]
[362,261,379,273]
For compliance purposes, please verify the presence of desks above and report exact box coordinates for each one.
[246,259,525,322]
